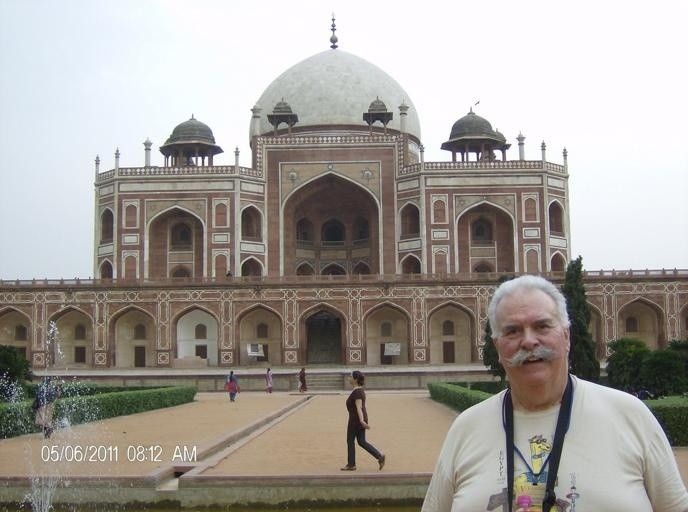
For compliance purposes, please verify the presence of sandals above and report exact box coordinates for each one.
[340,464,356,471]
[378,454,385,470]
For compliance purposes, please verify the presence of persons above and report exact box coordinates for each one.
[225,371,240,401]
[340,371,386,471]
[32,377,60,439]
[419,274,688,512]
[299,368,307,392]
[629,385,662,400]
[265,368,273,393]
[225,377,237,401]
